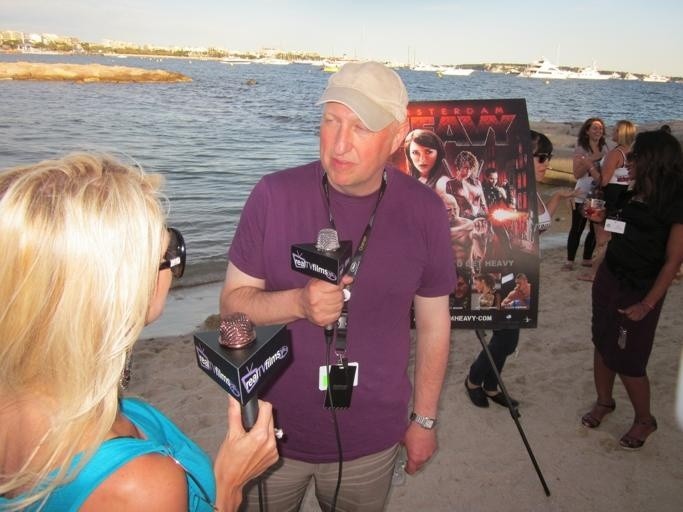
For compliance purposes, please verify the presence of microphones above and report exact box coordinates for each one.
[290,229,352,346]
[194,311,293,432]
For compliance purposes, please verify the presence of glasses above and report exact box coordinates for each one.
[533,154,552,164]
[159,228,186,278]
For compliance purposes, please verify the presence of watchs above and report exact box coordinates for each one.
[410,412,436,431]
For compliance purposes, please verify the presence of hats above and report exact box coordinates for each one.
[314,62,409,132]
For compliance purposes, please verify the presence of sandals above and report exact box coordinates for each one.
[619,416,657,448]
[582,400,615,428]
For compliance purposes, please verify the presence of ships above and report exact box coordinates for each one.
[505,57,669,83]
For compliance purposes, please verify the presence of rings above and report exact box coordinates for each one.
[274,428,282,439]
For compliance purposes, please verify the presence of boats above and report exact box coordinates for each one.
[410,63,438,72]
[538,157,577,187]
[294,59,409,74]
[439,67,474,77]
[116,55,127,59]
[251,57,290,66]
[219,54,251,66]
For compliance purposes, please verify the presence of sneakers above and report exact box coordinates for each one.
[487,392,519,407]
[465,375,489,408]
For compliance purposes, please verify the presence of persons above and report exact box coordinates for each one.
[660,124,670,134]
[580,130,682,452]
[465,130,584,407]
[402,128,531,311]
[0,153,280,512]
[560,118,634,281]
[218,60,453,512]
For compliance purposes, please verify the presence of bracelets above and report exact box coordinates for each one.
[641,301,654,310]
